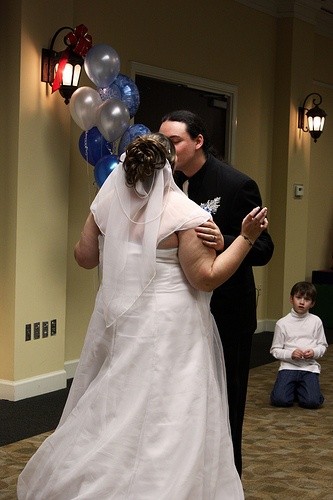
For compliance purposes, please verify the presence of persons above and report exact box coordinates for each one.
[269,279,327,408]
[61,133,268,500]
[158,111,273,477]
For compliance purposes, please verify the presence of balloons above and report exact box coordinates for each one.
[79,126,114,165]
[92,82,122,103]
[94,154,121,188]
[118,124,153,162]
[114,74,140,119]
[84,44,121,85]
[95,98,131,144]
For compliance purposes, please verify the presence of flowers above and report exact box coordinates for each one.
[200,197,220,215]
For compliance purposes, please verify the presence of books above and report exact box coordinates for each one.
[70,87,102,130]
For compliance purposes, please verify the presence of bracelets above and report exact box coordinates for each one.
[240,233,253,247]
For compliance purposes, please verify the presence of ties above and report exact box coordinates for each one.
[182,180,189,198]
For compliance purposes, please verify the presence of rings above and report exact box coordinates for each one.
[212,235,216,242]
[254,218,259,223]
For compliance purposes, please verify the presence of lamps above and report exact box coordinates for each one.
[298,93,328,143]
[41,26,84,105]
